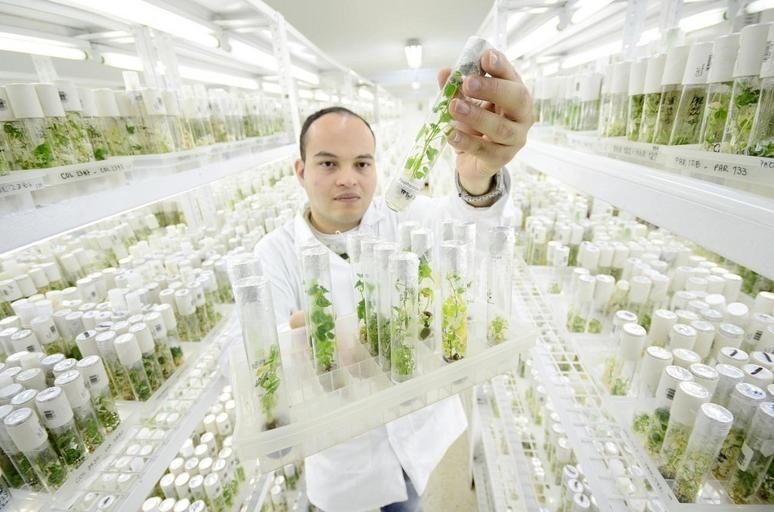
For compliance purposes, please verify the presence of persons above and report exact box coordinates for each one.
[224,49,537,510]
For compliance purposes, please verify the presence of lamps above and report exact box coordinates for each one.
[496,0,773,47]
[1,0,221,51]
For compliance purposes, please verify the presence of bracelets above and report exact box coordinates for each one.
[453,163,503,202]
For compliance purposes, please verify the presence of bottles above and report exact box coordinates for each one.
[4,86,306,510]
[352,241,424,385]
[296,245,345,391]
[307,87,404,173]
[384,35,494,215]
[489,24,774,511]
[397,221,474,365]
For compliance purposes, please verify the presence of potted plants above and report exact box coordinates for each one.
[0,24,773,512]
